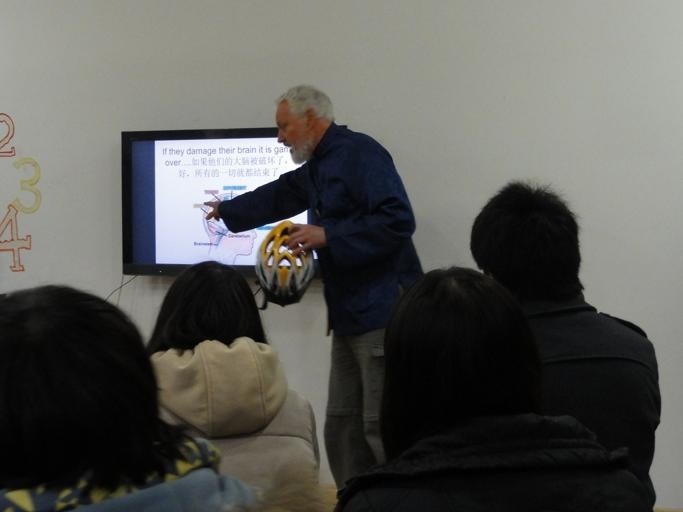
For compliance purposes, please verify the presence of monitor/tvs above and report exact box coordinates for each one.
[121,127,325,281]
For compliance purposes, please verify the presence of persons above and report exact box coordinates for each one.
[0,282,258,510]
[329,265,649,511]
[202,83,424,489]
[146,257,320,511]
[468,179,661,476]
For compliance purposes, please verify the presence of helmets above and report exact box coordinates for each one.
[254,221,315,307]
